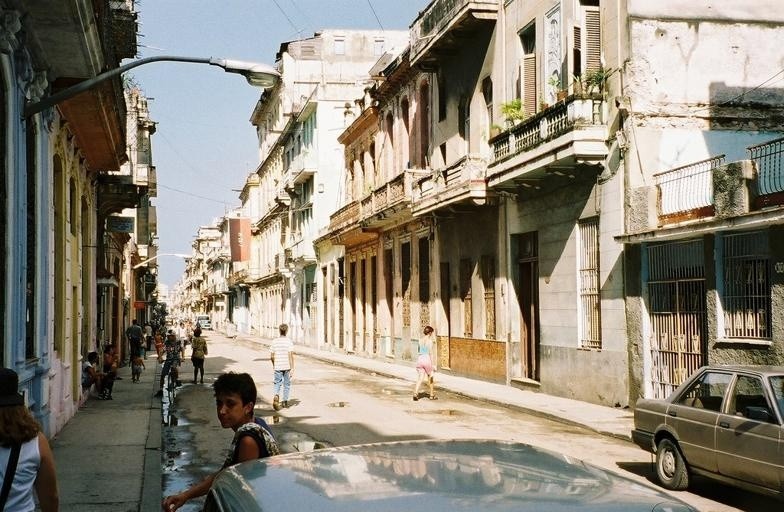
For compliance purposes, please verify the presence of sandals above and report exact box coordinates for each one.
[413,393,418,401]
[430,395,438,400]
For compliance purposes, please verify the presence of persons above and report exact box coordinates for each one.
[155,329,186,398]
[81,345,124,400]
[413,326,439,401]
[269,324,294,411]
[161,371,282,512]
[132,355,146,381]
[0,367,60,512]
[191,326,209,384]
[126,319,202,362]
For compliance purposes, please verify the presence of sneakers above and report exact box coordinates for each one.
[273,395,280,410]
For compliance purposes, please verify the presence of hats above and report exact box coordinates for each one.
[165,329,177,336]
[0,368,23,405]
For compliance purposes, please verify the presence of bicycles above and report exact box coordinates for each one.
[157,355,182,406]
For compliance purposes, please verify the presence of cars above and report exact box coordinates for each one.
[204,439,686,511]
[194,314,213,331]
[165,318,173,325]
[630,364,783,505]
